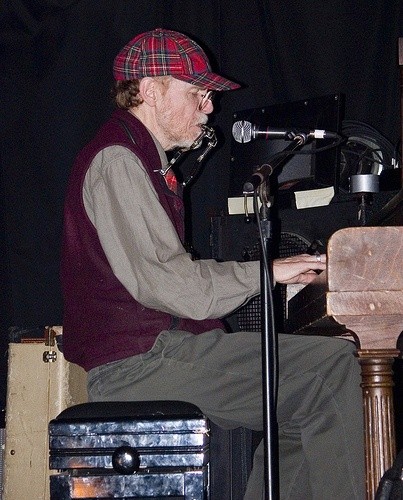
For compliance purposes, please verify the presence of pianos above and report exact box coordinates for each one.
[273,227,403,500]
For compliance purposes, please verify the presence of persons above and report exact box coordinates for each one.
[57,27,367,500]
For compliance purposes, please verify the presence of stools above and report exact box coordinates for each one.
[49,401,214,500]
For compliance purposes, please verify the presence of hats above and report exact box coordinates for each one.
[113,28,240,91]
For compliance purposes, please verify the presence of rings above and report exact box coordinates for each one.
[316,254,321,263]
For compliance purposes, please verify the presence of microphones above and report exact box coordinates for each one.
[231,120,338,142]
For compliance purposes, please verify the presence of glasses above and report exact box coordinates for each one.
[192,91,213,112]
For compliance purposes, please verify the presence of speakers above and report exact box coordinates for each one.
[208,188,402,332]
